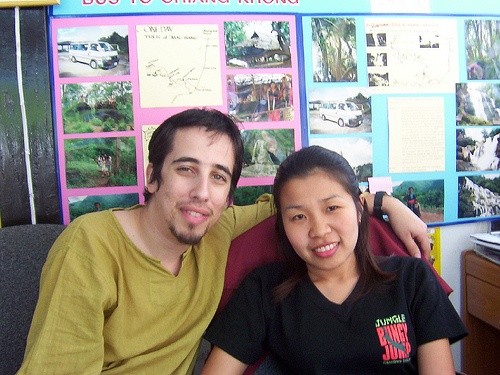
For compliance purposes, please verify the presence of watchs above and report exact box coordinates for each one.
[373,190,392,221]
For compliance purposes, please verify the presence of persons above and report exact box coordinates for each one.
[406,185,416,211]
[200,144,468,375]
[435,193,440,209]
[266,82,281,111]
[261,130,279,165]
[14,105,430,375]
[99,152,113,176]
[414,198,422,215]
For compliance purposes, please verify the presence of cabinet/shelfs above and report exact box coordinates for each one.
[457,249,500,375]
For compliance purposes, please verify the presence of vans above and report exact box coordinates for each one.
[69,41,119,71]
[319,101,364,129]
